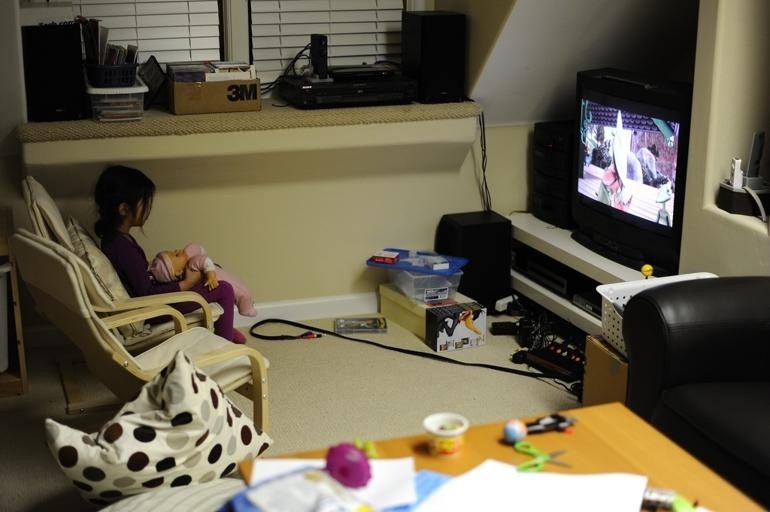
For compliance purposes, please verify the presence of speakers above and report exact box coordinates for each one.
[402,12,466,103]
[526,122,572,230]
[435,210,513,315]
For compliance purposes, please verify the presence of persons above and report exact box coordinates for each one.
[91,163,250,345]
[590,132,612,169]
[634,143,669,189]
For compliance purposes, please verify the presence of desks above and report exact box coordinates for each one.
[237,400,770,512]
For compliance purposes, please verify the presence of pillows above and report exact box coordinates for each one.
[43,349,274,510]
[66,213,151,341]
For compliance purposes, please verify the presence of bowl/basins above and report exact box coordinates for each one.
[422,412,469,457]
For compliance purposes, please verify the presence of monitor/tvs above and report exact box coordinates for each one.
[571,75,691,278]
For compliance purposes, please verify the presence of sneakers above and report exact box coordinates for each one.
[232,324,247,344]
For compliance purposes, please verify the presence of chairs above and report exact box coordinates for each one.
[8,227,272,436]
[622,276,770,512]
[21,175,225,416]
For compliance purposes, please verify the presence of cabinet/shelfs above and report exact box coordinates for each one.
[507,211,667,338]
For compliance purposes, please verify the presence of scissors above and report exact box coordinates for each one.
[515,442,572,472]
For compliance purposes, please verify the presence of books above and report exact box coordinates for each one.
[78,14,257,123]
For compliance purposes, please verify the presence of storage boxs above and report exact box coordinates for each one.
[85,74,150,123]
[84,62,139,88]
[379,283,478,343]
[581,334,629,407]
[366,247,470,305]
[166,73,261,115]
